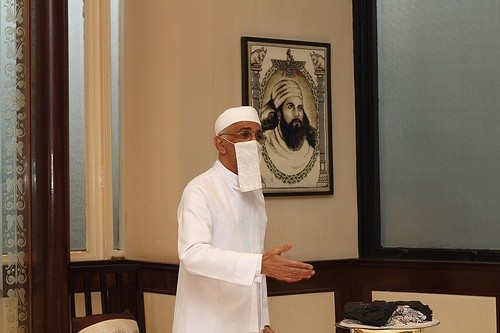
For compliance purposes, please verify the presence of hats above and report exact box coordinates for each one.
[272,79,303,110]
[213,106,261,137]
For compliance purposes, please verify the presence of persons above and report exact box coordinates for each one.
[169,106,316,333]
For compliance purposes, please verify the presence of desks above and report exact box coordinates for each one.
[334,314,440,333]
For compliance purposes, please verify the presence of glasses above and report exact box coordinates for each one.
[221,131,263,141]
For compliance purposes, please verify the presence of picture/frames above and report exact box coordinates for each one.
[240,36,335,197]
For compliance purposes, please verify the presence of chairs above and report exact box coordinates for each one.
[67,259,147,333]
